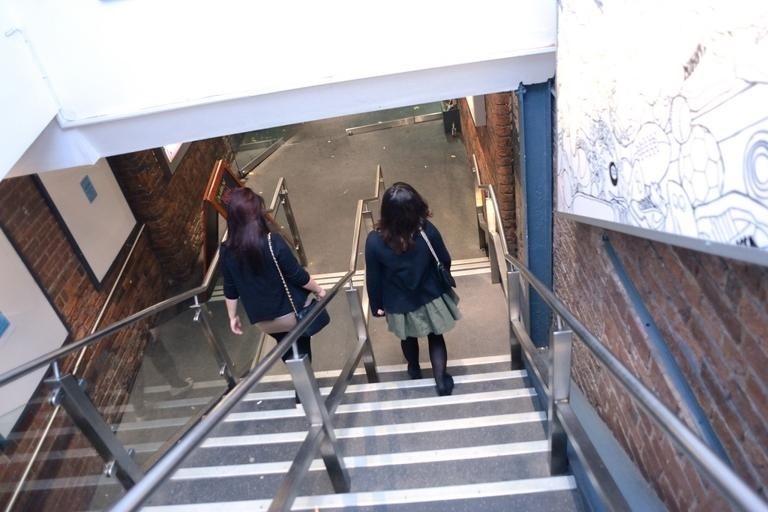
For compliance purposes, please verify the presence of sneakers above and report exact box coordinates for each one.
[442,371,455,395]
[406,366,423,380]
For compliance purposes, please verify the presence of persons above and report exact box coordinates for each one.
[217,187,328,411]
[364,180,461,397]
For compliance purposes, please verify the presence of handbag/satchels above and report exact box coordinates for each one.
[433,260,457,291]
[294,298,332,338]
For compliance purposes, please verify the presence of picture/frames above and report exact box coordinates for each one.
[0,227,69,444]
[32,158,137,291]
[152,143,191,177]
